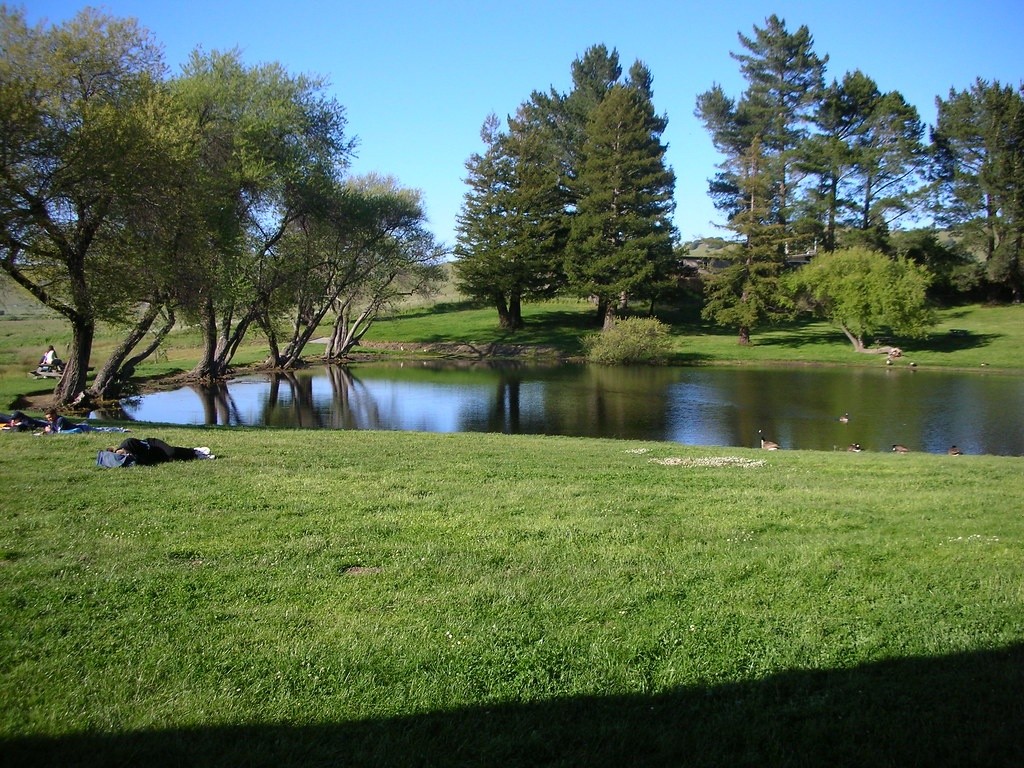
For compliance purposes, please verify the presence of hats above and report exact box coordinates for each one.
[10,411,23,420]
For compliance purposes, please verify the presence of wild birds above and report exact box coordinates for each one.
[948,446,963,456]
[839,412,849,424]
[891,445,910,454]
[758,430,780,450]
[847,443,864,452]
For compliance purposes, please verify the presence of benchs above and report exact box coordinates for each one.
[30,369,63,379]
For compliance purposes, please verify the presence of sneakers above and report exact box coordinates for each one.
[194,447,215,459]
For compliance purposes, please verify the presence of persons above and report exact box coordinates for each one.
[44,409,132,434]
[10,411,51,427]
[107,437,216,467]
[39,345,67,372]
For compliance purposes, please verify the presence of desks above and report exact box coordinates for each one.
[37,362,67,371]
